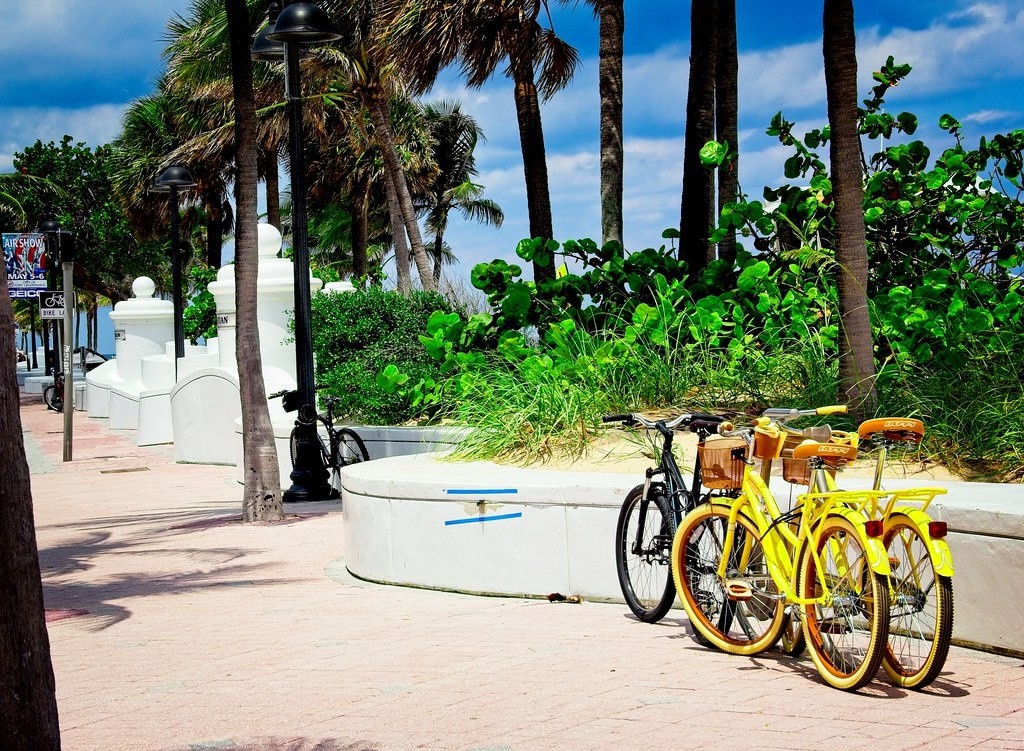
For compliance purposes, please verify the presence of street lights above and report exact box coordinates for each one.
[148,167,200,359]
[38,220,64,411]
[254,3,345,502]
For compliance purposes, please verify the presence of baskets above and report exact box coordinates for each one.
[697,438,747,489]
[281,395,298,412]
[783,436,837,485]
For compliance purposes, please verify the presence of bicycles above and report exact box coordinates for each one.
[43,372,65,415]
[269,382,372,496]
[603,403,955,690]
